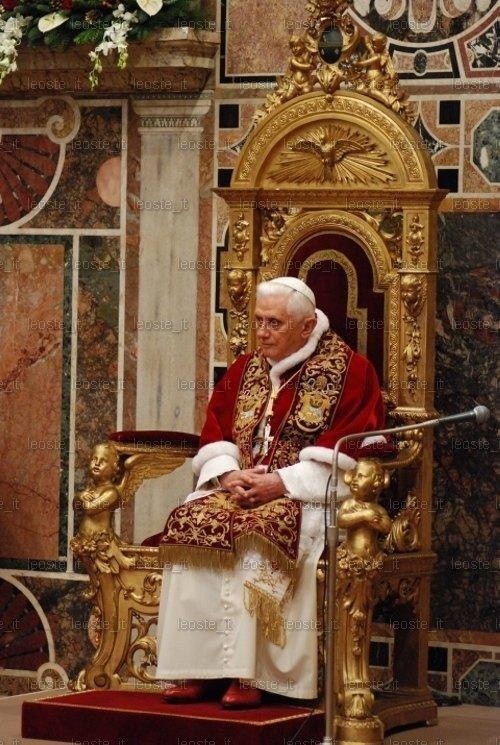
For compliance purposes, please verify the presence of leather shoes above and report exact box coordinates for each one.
[221,678,269,707]
[164,679,215,704]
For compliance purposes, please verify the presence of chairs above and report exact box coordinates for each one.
[67,1,447,745]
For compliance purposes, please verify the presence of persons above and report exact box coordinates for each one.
[70,440,121,601]
[153,276,394,708]
[400,274,423,314]
[280,32,319,104]
[226,269,250,309]
[350,32,400,114]
[336,457,392,623]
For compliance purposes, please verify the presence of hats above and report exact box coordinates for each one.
[267,276,316,311]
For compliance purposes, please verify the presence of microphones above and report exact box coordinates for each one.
[439,405,490,426]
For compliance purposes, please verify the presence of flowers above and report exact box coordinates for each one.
[0,0,219,95]
